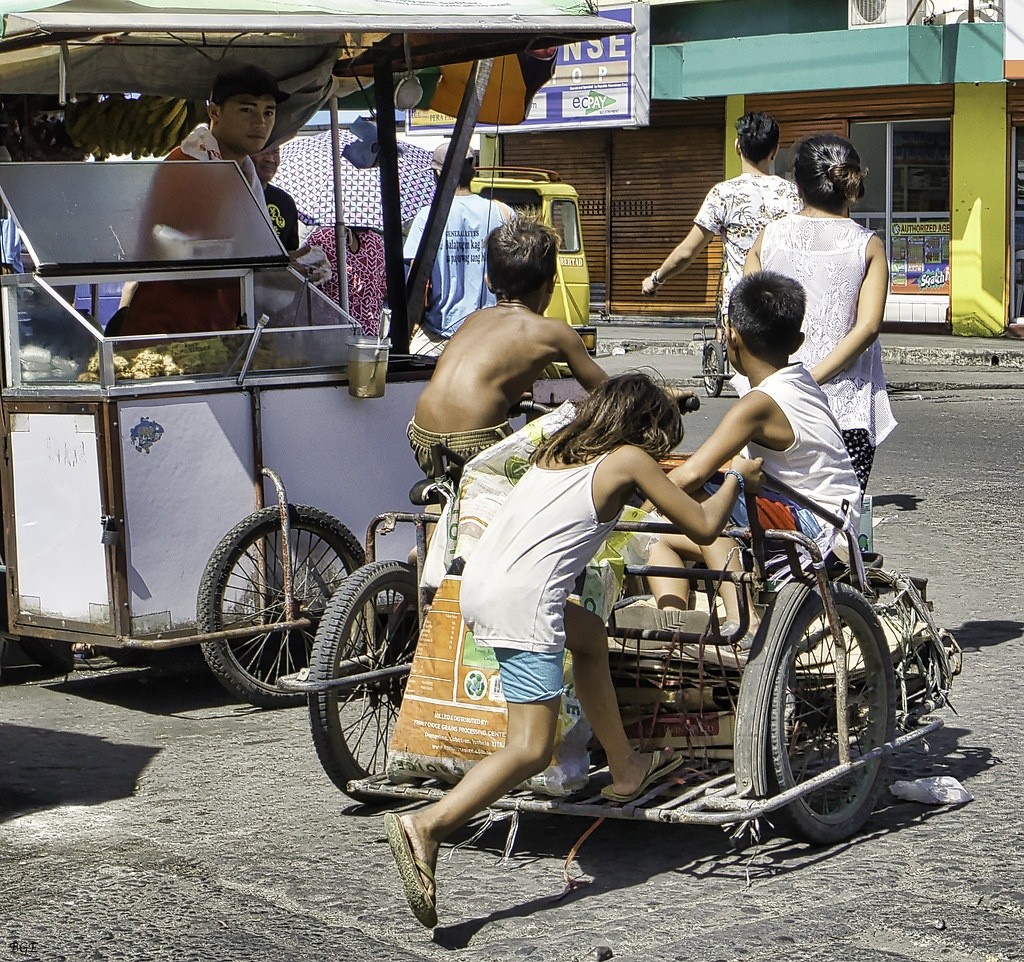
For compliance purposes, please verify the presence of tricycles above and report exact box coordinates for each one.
[276,390,964,844]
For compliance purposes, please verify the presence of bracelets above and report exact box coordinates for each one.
[725,471,745,494]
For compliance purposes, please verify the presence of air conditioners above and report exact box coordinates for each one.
[848,0,926,30]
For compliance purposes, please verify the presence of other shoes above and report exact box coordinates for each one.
[407,503,442,564]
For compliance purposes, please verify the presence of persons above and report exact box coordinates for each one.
[112,62,278,351]
[384,363,766,928]
[299,223,388,340]
[403,140,519,358]
[727,132,899,499]
[639,110,802,347]
[249,145,300,252]
[0,211,26,300]
[406,214,699,566]
[637,271,863,670]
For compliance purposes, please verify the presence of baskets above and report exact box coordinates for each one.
[393,75,423,111]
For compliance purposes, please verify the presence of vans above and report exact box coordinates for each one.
[467,162,599,363]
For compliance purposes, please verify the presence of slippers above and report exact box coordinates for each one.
[608,600,711,650]
[599,750,685,802]
[383,812,438,928]
[704,621,755,657]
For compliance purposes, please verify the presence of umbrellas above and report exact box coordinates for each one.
[268,126,439,230]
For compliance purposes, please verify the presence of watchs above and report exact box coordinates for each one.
[650,270,666,285]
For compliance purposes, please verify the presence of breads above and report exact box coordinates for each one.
[76,335,306,383]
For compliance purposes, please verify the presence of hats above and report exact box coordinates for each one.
[420,141,477,172]
[212,64,291,104]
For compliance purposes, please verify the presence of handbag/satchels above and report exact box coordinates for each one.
[291,245,332,286]
